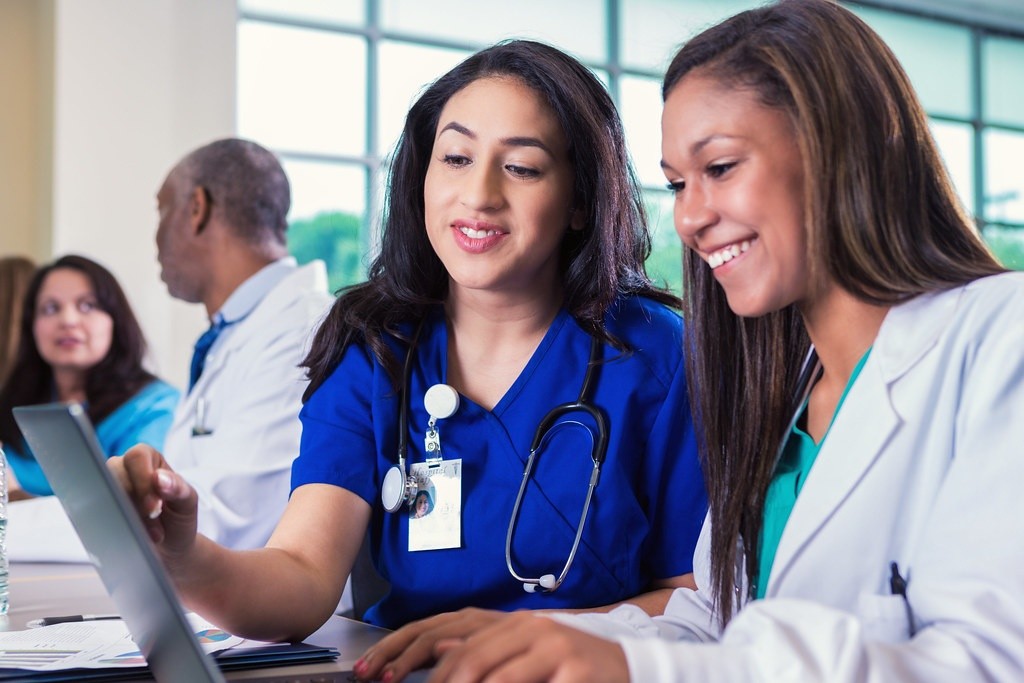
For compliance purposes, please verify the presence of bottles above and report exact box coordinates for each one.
[0,448,11,616]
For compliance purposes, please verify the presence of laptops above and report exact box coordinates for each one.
[11,401,437,683]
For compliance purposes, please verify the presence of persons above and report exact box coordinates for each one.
[0,255,181,502]
[105,40,707,643]
[352,0,1024,683]
[155,138,353,616]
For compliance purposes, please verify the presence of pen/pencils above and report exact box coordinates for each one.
[891,563,907,594]
[196,399,205,434]
[26,615,122,628]
